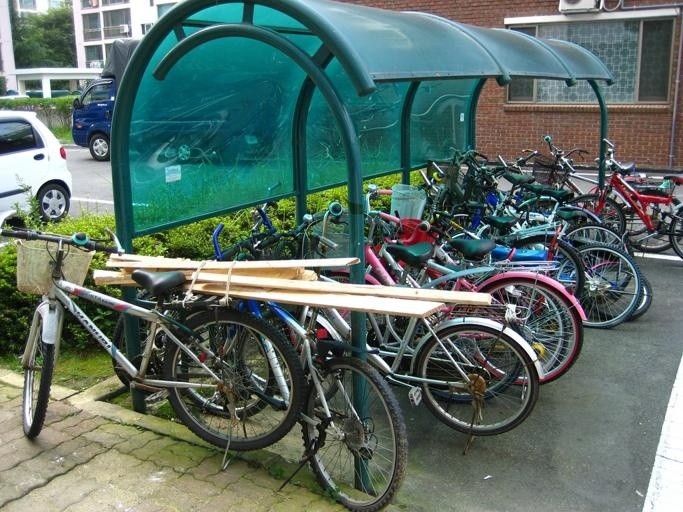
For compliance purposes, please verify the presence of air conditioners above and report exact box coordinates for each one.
[558,0,599,14]
[120,24,129,33]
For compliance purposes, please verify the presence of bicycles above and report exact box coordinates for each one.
[111,134,683,455]
[161,207,408,512]
[4,226,303,459]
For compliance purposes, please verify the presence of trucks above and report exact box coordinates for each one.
[64,37,287,162]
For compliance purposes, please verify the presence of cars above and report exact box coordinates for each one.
[0,106,73,230]
